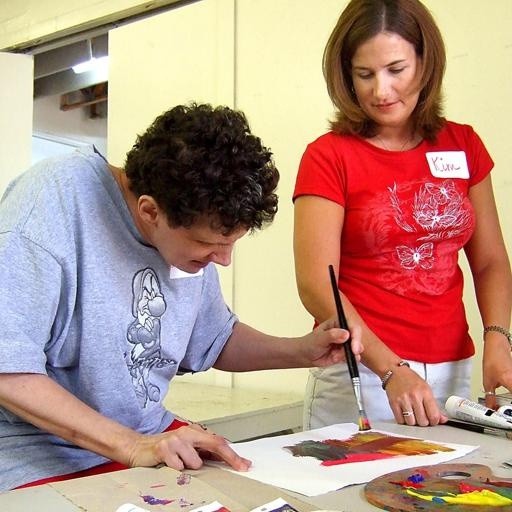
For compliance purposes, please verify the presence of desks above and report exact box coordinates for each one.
[160,378,304,444]
[2,391,511,510]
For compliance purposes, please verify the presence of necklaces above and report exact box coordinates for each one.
[378,134,413,150]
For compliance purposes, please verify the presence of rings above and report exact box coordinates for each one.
[484,390,496,398]
[402,410,413,418]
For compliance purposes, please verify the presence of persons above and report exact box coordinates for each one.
[0,99,366,493]
[290,0,512,436]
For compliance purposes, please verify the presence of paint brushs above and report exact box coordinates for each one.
[444,420,512,441]
[328,265,371,431]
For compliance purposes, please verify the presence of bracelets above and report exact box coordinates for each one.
[380,359,411,392]
[484,324,512,352]
